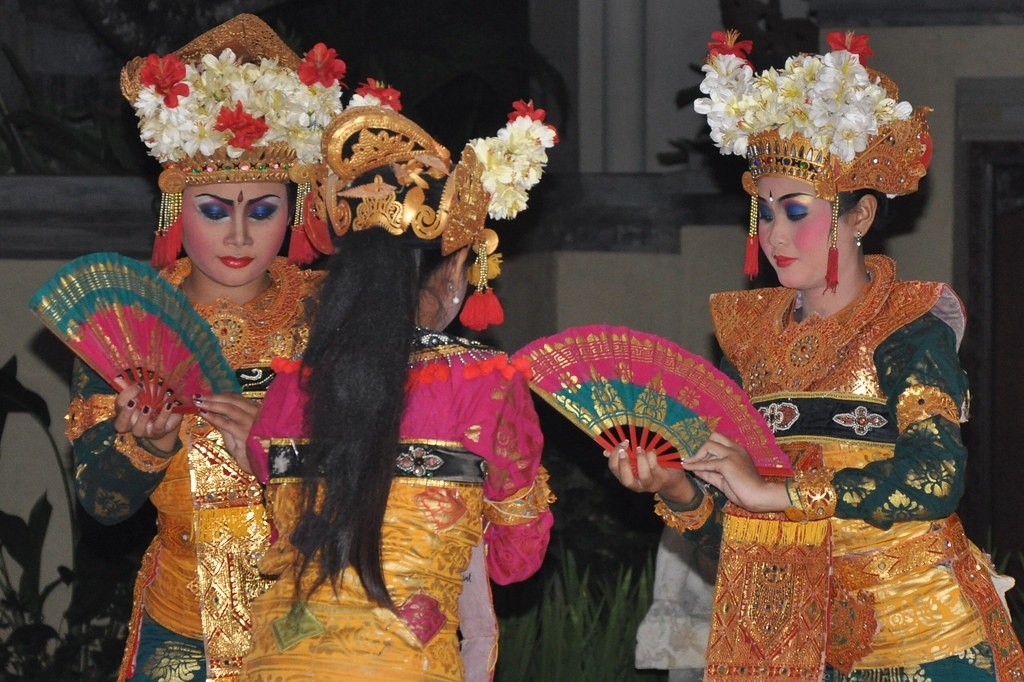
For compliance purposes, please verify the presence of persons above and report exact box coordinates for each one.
[603,30,1024,682]
[236,79,558,682]
[70,12,331,682]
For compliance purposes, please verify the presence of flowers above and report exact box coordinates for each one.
[130,43,346,165]
[352,80,402,117]
[694,26,913,160]
[468,98,559,221]
